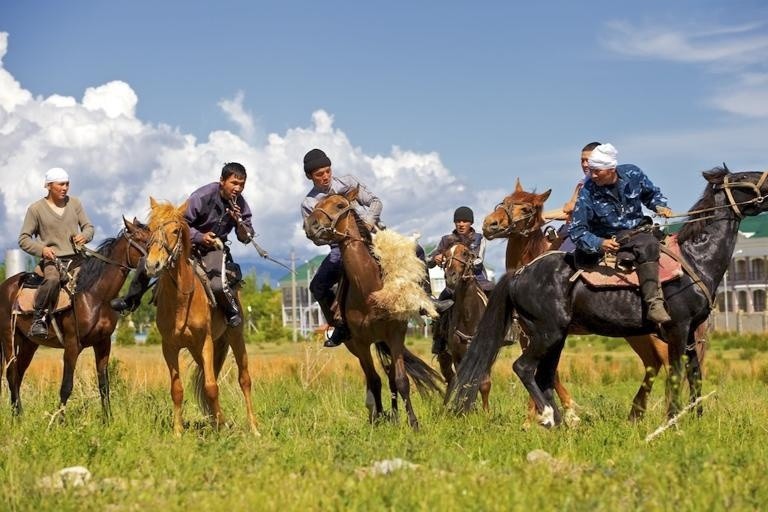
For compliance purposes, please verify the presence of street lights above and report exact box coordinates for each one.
[302,259,313,331]
[723,228,757,340]
[729,248,743,260]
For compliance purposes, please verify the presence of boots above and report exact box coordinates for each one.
[419,254,455,316]
[26,309,49,337]
[635,261,672,322]
[431,322,441,354]
[319,290,352,347]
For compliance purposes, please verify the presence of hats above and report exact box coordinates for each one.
[588,143,618,170]
[45,167,68,184]
[304,149,331,173]
[454,208,474,225]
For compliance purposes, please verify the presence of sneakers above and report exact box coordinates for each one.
[229,314,241,328]
[110,296,140,312]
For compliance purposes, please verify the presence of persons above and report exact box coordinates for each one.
[110,163,255,328]
[424,207,513,354]
[18,168,95,337]
[301,148,454,347]
[568,145,672,323]
[542,142,602,252]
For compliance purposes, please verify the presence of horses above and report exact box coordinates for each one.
[303,181,446,433]
[441,161,768,429]
[482,175,705,430]
[144,196,262,438]
[431,232,492,415]
[0,212,152,427]
